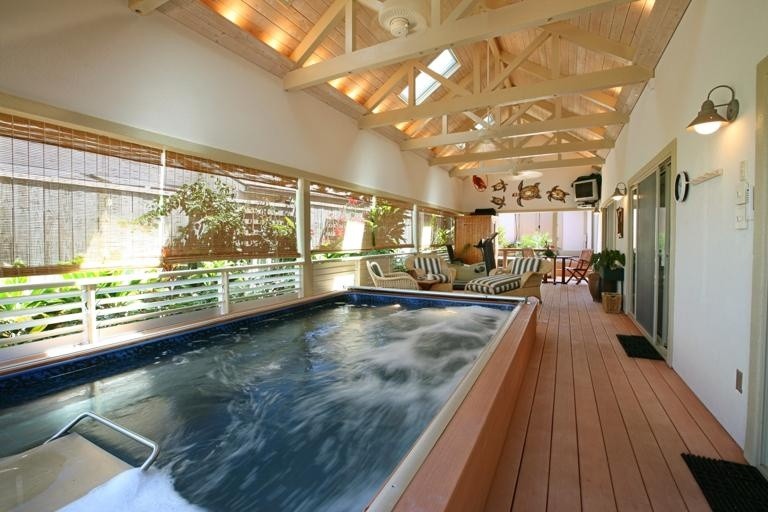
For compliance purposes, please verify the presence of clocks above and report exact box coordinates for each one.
[674,172,689,203]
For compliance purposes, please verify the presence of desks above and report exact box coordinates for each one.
[417,279,440,290]
[541,256,572,285]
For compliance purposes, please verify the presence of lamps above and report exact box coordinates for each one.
[610,182,627,201]
[377,8,416,36]
[686,85,739,135]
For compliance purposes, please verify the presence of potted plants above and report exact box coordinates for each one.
[587,248,625,303]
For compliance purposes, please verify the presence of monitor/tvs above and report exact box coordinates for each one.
[573,178,599,202]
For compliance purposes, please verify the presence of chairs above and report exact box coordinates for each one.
[521,247,536,257]
[562,249,594,284]
[405,254,456,290]
[366,260,418,290]
[464,257,553,304]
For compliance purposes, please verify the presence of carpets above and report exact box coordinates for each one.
[616,335,665,361]
[681,452,768,512]
